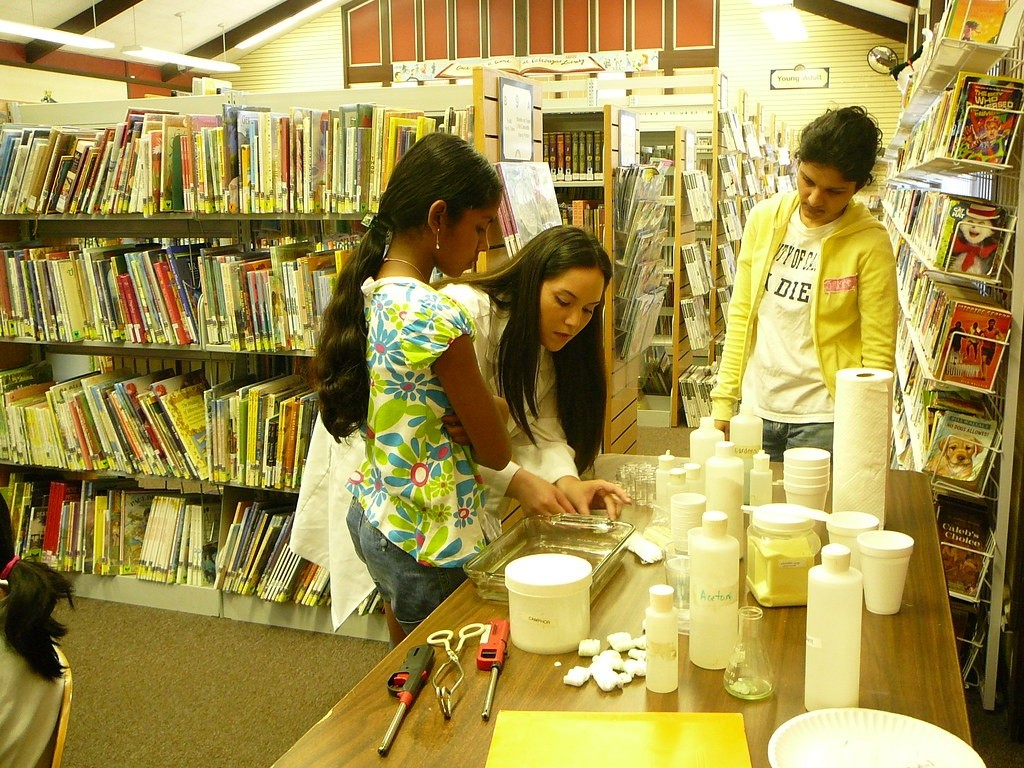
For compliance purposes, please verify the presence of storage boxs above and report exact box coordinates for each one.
[463,513,636,608]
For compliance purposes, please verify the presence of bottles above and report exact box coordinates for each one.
[644,401,777,699]
[804,543,863,713]
[745,503,821,609]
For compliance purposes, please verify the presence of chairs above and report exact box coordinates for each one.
[33,644,72,768]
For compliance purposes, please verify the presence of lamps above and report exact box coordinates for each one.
[0,0,116,49]
[121,4,240,72]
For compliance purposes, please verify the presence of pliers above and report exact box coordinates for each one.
[427,622,485,719]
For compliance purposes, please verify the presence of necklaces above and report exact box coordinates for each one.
[382,259,429,285]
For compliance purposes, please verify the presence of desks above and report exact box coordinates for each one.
[265,453,974,768]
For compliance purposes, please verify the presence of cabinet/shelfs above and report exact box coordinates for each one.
[880,1,1024,712]
[0,67,713,641]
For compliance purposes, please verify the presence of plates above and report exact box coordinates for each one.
[767,709,987,768]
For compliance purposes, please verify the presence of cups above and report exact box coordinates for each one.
[663,541,690,635]
[670,492,707,556]
[783,448,831,510]
[504,552,593,655]
[857,530,914,615]
[826,510,879,572]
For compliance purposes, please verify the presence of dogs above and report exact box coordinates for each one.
[927,434,984,479]
[887,27,934,110]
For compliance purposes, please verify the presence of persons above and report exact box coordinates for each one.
[290,225,613,633]
[946,321,968,353]
[0,491,75,768]
[966,322,984,361]
[315,132,512,649]
[710,105,898,462]
[979,320,1004,380]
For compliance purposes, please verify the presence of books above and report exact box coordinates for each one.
[543,109,799,429]
[868,0,1024,667]
[0,75,564,617]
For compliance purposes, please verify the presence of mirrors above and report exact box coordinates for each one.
[867,45,898,74]
